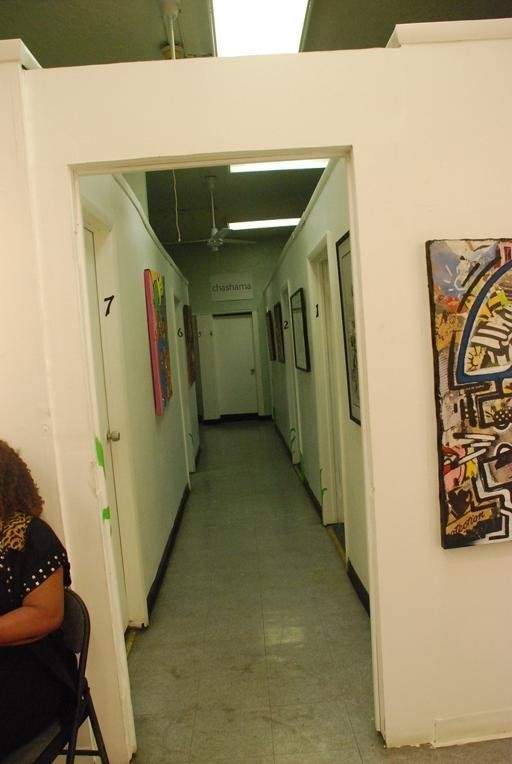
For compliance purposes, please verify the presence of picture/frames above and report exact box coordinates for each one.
[291,287,313,373]
[334,227,365,427]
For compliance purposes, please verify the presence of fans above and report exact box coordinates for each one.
[162,176,260,258]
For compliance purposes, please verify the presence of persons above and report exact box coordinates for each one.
[0,440,81,757]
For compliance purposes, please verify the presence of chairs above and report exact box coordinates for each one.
[2,585,111,762]
